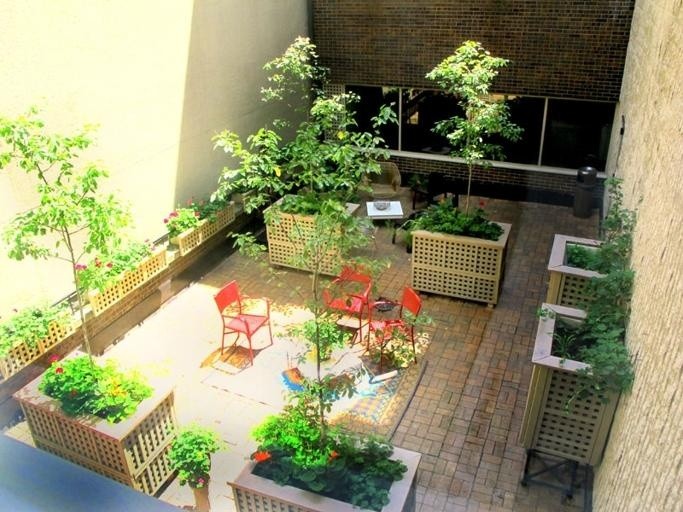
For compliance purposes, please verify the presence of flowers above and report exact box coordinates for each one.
[163,192,228,238]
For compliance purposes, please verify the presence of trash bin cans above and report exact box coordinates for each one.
[573,167,596,218]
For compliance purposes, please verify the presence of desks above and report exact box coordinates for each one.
[365,201,403,244]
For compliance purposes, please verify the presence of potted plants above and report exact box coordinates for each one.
[210,36,398,278]
[171,429,222,498]
[407,40,523,306]
[227,136,433,511]
[519,174,635,467]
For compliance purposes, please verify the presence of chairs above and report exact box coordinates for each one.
[213,272,421,370]
[360,161,401,201]
